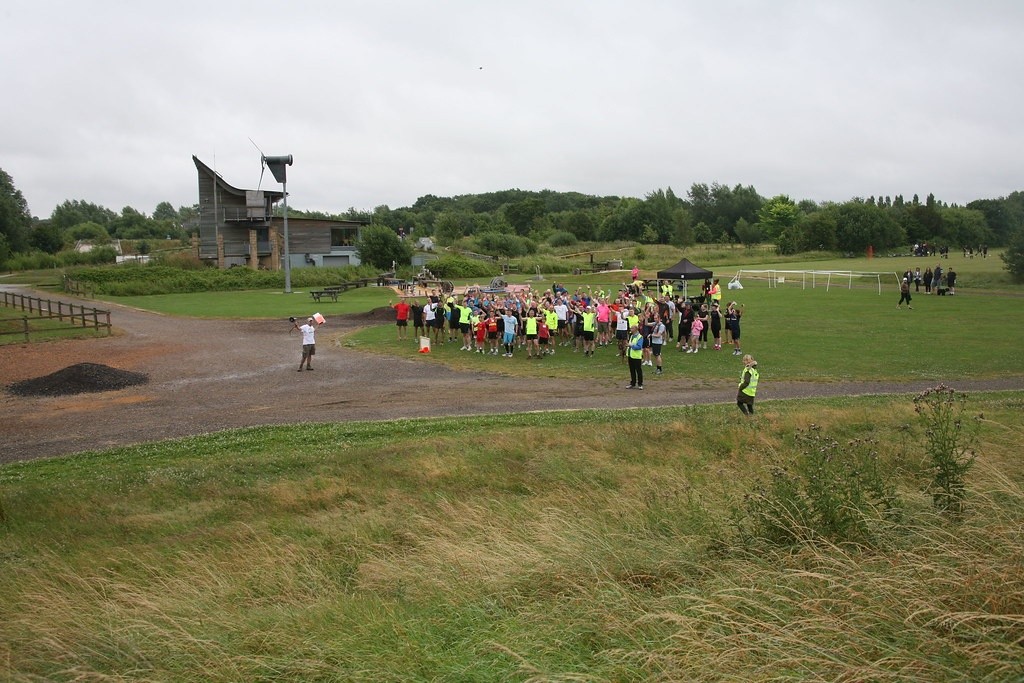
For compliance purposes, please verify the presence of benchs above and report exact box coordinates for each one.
[310,278,371,302]
[619,278,657,292]
[384,279,443,295]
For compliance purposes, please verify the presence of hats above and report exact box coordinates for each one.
[903,278,907,280]
[307,318,313,321]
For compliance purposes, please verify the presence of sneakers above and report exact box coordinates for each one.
[733,348,742,355]
[626,384,643,390]
[713,345,721,350]
[642,361,652,366]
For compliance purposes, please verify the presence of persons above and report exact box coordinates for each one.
[388,281,745,388]
[895,265,958,310]
[631,265,639,281]
[735,354,759,413]
[293,316,325,371]
[911,240,989,259]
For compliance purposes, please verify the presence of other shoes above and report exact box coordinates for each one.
[896,306,901,309]
[916,291,955,295]
[298,368,302,371]
[723,340,733,344]
[652,369,661,375]
[307,366,314,370]
[663,340,707,354]
[399,336,615,360]
[909,307,913,310]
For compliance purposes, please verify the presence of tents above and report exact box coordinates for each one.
[653,258,712,302]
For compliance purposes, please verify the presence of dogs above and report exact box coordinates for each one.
[937,288,949,296]
